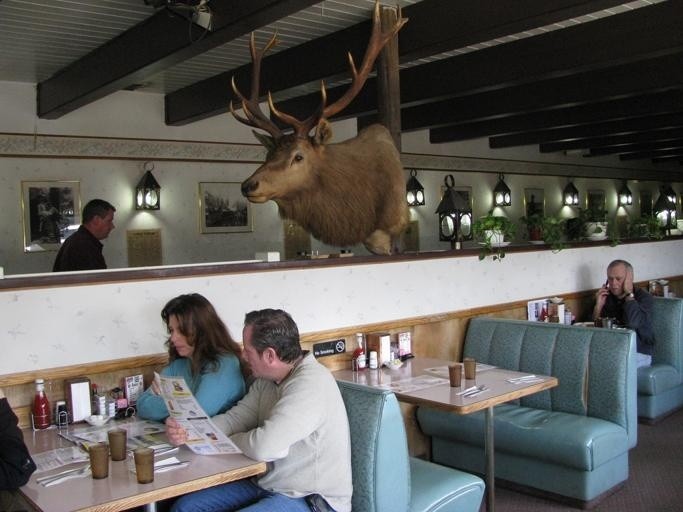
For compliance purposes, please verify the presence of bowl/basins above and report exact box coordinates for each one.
[84,414,109,425]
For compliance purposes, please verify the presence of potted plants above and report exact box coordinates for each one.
[671,215,683,233]
[626,211,662,241]
[471,216,518,262]
[519,213,565,252]
[576,210,620,246]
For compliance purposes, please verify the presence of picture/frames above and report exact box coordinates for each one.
[585,187,607,211]
[19,178,86,257]
[194,177,255,234]
[439,186,473,213]
[399,221,419,252]
[637,189,653,217]
[519,184,546,219]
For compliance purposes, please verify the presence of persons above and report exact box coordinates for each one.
[0,387,36,490]
[51,197,117,271]
[135,294,246,426]
[591,260,657,369]
[167,307,353,512]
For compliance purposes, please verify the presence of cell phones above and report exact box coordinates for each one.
[116,407,135,420]
[605,279,609,289]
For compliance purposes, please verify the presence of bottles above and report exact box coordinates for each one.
[31,378,50,429]
[649,282,656,296]
[90,383,100,414]
[57,406,67,424]
[351,332,367,370]
[55,400,64,423]
[539,304,548,322]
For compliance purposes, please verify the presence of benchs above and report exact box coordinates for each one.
[412,316,641,510]
[634,292,683,422]
[335,380,484,512]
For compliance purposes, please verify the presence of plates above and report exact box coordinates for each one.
[575,322,594,326]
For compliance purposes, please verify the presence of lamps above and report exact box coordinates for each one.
[655,194,678,239]
[434,173,474,242]
[405,167,426,208]
[617,177,633,207]
[146,1,220,39]
[132,160,163,213]
[561,174,581,208]
[492,173,512,207]
[659,178,676,205]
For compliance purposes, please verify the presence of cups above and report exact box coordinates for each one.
[592,317,603,328]
[447,363,463,388]
[462,358,477,380]
[131,447,156,485]
[87,442,110,479]
[602,318,612,329]
[106,427,128,462]
[550,316,559,323]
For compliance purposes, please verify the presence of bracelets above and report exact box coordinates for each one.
[624,291,634,299]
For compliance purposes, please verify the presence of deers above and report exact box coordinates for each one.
[228,0,411,257]
